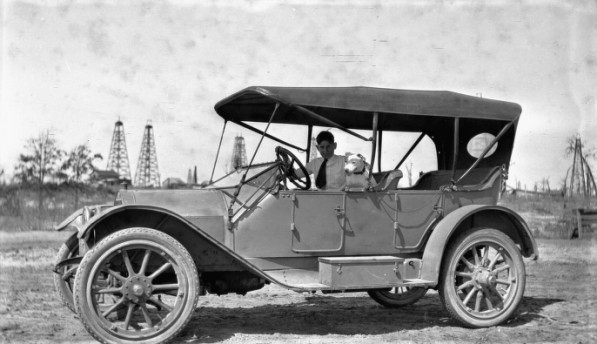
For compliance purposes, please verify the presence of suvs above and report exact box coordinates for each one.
[53,86,539,344]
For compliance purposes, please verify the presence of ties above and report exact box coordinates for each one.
[315,157,328,189]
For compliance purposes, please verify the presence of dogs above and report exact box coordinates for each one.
[344,152,378,192]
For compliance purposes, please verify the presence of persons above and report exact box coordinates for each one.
[294,131,348,192]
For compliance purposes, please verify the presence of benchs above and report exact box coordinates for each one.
[402,166,501,190]
[371,169,403,190]
